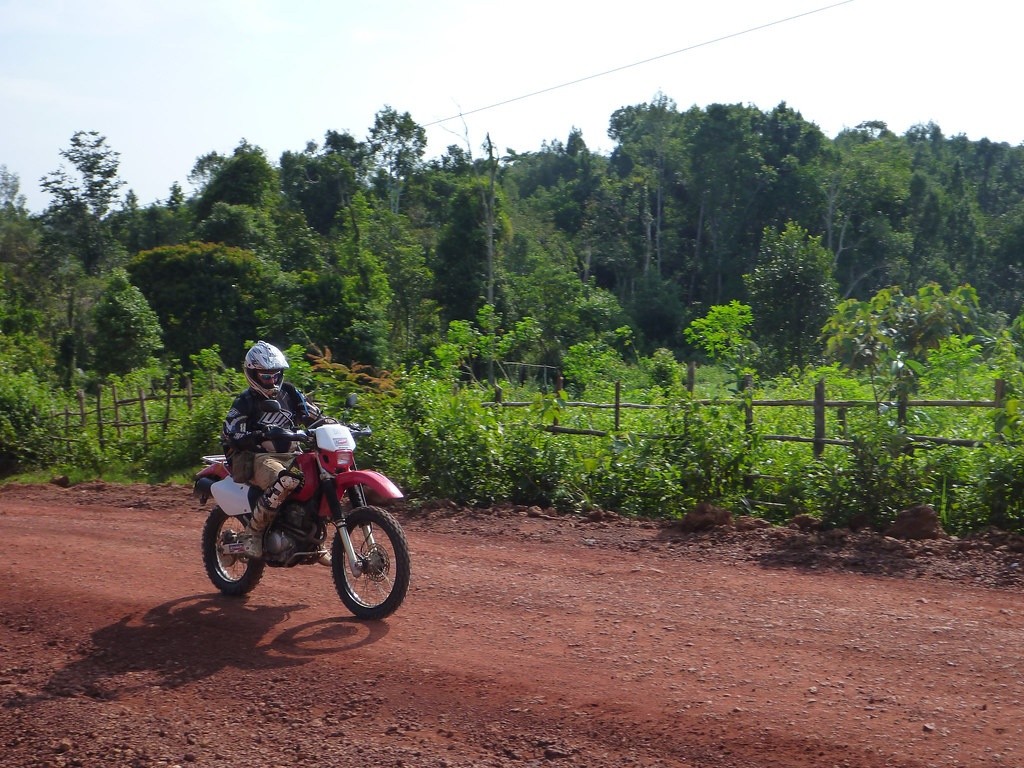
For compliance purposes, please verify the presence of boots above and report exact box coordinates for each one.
[244,501,277,559]
[317,545,333,566]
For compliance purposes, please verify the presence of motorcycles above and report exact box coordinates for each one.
[193,393,412,622]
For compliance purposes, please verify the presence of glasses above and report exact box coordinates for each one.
[257,370,281,381]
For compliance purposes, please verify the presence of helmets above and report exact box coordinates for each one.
[244,341,289,400]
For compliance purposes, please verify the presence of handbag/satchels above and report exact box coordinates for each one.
[232,449,254,483]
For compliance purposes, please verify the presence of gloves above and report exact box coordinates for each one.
[347,423,360,432]
[265,424,282,440]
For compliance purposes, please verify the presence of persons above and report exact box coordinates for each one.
[222,340,361,560]
